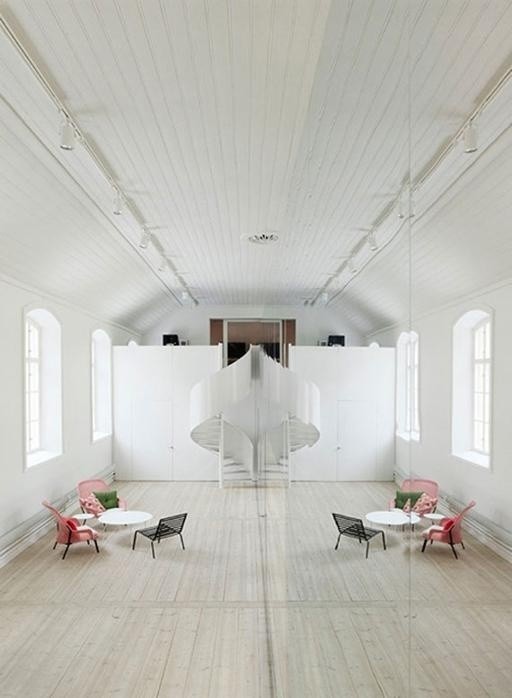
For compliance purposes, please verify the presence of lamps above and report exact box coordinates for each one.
[56,113,76,153]
[461,120,480,154]
[367,196,416,252]
[110,192,150,250]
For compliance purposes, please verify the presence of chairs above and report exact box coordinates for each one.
[132,513,189,558]
[387,478,477,560]
[41,479,126,561]
[331,512,385,559]
